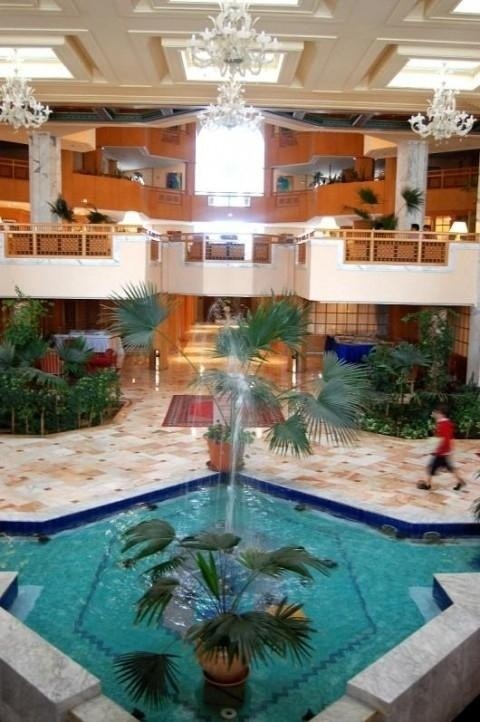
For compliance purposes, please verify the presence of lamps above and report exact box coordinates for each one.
[181,11,280,132]
[313,217,340,238]
[0,49,54,132]
[449,220,469,241]
[407,53,476,145]
[116,211,145,234]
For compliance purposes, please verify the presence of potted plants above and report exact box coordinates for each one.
[112,511,339,714]
[201,423,258,473]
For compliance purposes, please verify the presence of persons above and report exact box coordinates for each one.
[417,405,468,490]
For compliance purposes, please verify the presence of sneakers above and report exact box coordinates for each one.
[417,481,466,490]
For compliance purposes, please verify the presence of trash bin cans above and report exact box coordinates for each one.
[291,354,298,373]
[154,349,160,371]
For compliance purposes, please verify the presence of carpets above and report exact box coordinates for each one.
[161,394,287,430]
